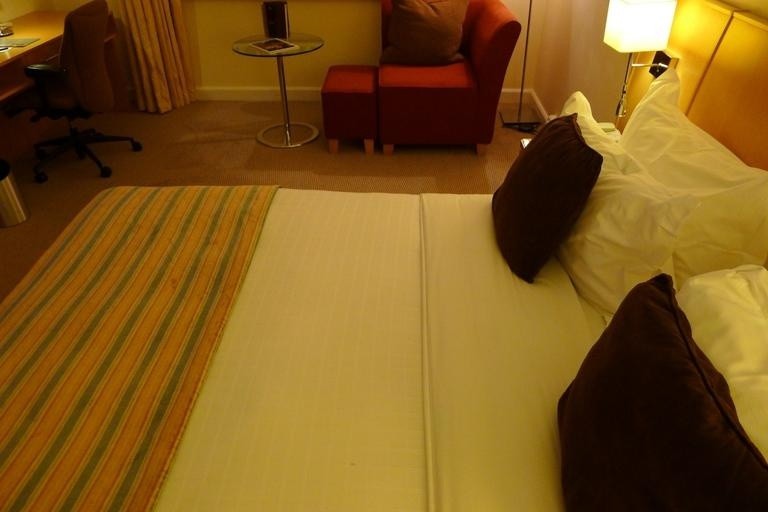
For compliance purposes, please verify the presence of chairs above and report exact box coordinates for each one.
[24,0,144,183]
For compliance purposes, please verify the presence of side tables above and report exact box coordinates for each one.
[231,32,325,149]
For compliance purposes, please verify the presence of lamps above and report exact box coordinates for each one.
[602,0,680,117]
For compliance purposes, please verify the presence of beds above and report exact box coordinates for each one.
[0,185,768,512]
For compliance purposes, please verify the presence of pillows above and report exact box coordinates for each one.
[379,0,471,65]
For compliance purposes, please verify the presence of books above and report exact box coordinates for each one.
[250,38,301,54]
[0,37,40,47]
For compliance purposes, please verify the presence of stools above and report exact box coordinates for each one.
[322,65,375,155]
[378,1,523,158]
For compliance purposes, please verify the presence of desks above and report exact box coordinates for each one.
[0,9,133,114]
